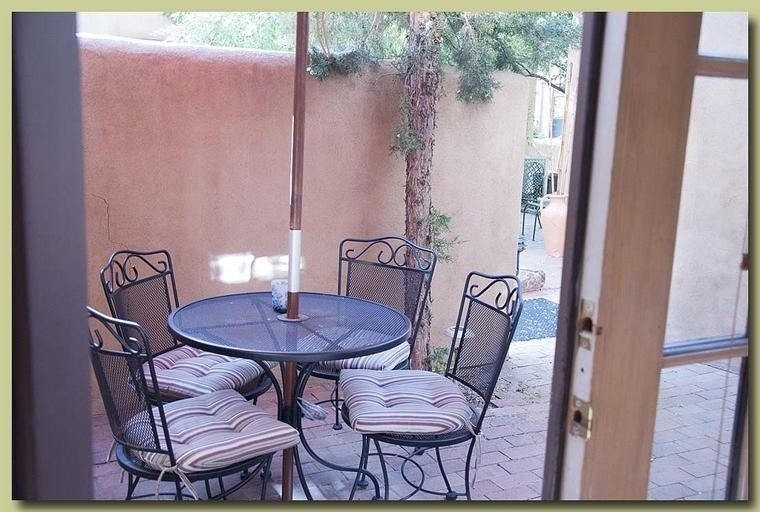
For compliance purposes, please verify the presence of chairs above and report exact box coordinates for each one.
[86,305,301,501]
[100,250,278,479]
[338,271,525,501]
[522,196,543,240]
[298,237,438,430]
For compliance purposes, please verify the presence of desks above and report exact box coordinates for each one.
[167,290,412,500]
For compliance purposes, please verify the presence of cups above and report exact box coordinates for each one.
[271,280,288,314]
